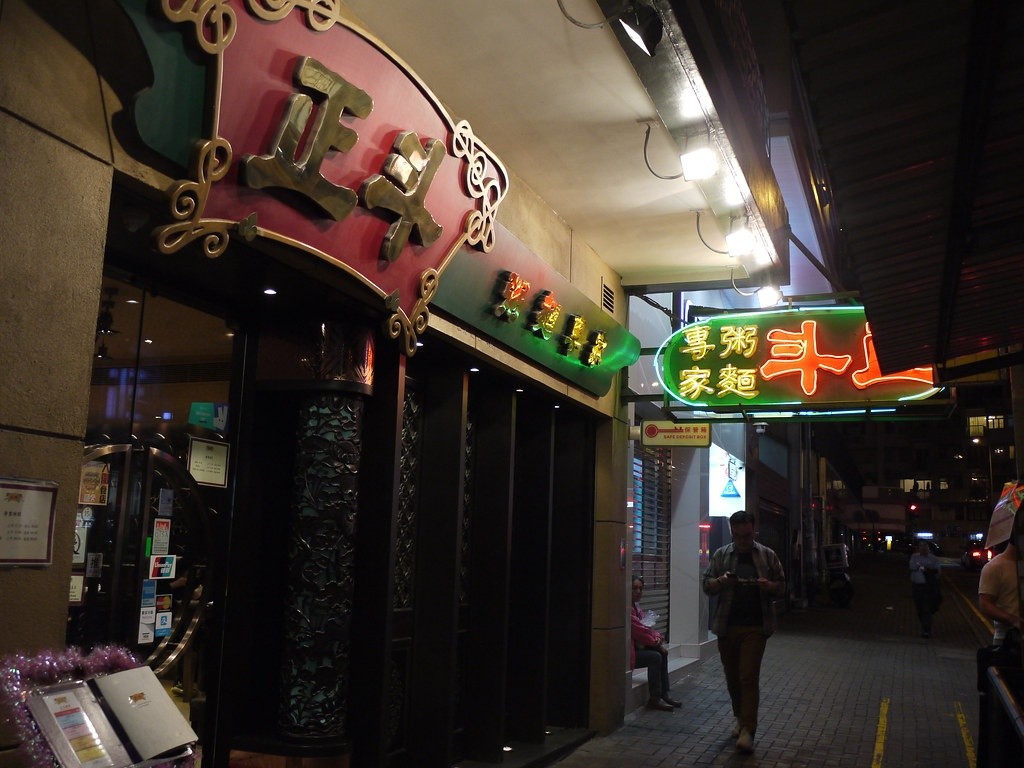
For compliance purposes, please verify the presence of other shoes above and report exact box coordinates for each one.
[663,696,682,708]
[647,698,673,711]
[922,631,930,638]
[731,717,741,737]
[736,728,754,753]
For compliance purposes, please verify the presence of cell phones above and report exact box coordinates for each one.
[726,573,737,579]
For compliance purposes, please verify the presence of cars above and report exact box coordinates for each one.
[959,540,995,567]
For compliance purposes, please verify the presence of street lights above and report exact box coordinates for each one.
[973,439,995,505]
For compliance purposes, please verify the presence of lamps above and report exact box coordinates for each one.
[637,118,719,182]
[95,287,119,361]
[557,0,663,56]
[689,209,757,257]
[727,265,783,308]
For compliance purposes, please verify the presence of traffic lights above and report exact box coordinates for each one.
[910,504,917,511]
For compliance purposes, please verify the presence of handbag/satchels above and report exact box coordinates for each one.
[977,627,1024,694]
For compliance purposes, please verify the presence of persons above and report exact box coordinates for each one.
[155,540,203,697]
[630,574,682,710]
[909,539,942,638]
[702,511,786,752]
[976,529,1024,647]
